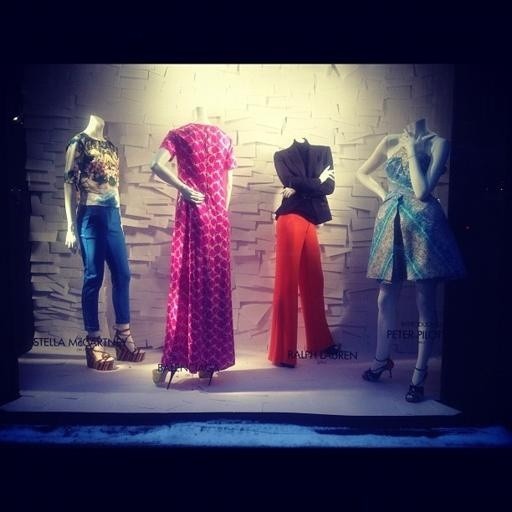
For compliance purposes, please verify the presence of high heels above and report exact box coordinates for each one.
[199,370,215,385]
[316,345,341,355]
[405,365,428,402]
[86,328,146,371]
[362,358,394,382]
[152,367,177,390]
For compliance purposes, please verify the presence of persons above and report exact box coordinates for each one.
[150,105,236,391]
[356,118,456,403]
[267,132,343,369]
[62,113,146,371]
